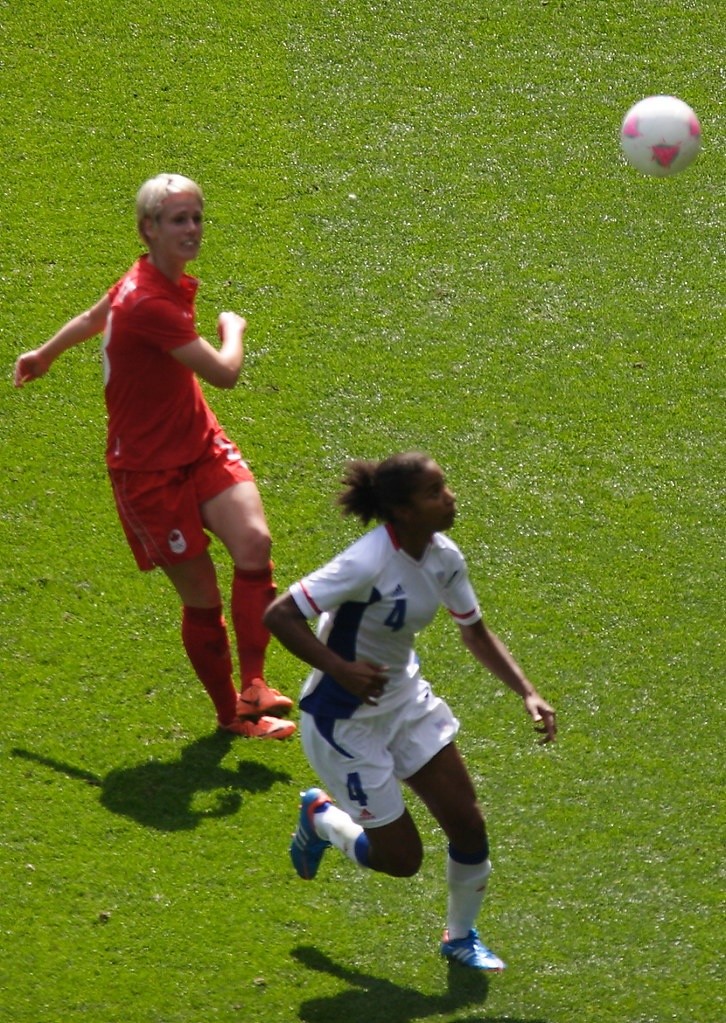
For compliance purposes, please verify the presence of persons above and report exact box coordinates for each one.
[9,171,297,744]
[256,451,562,972]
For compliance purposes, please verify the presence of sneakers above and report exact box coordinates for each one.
[291,788,333,880]
[237,677,294,724]
[441,929,507,971]
[222,692,295,740]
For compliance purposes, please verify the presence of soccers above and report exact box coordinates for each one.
[620,95,701,178]
